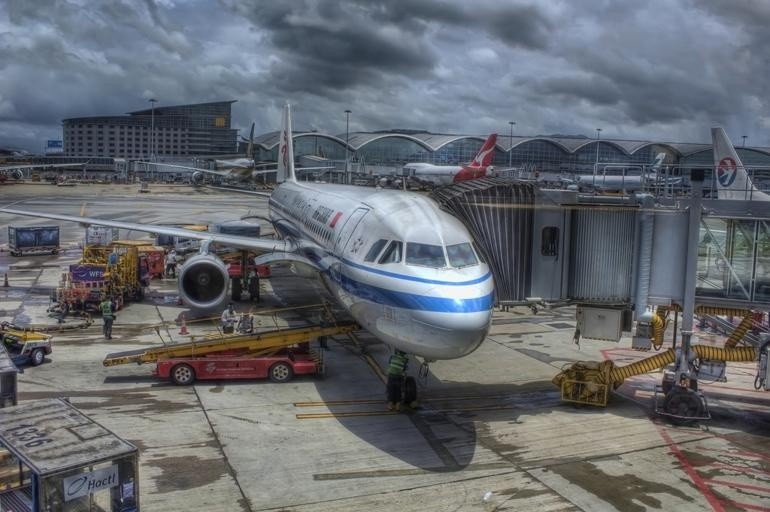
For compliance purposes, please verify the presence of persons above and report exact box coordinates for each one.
[248,268,259,303]
[222,302,238,334]
[164,245,178,279]
[317,307,330,350]
[57,300,69,323]
[385,350,408,412]
[99,295,116,339]
